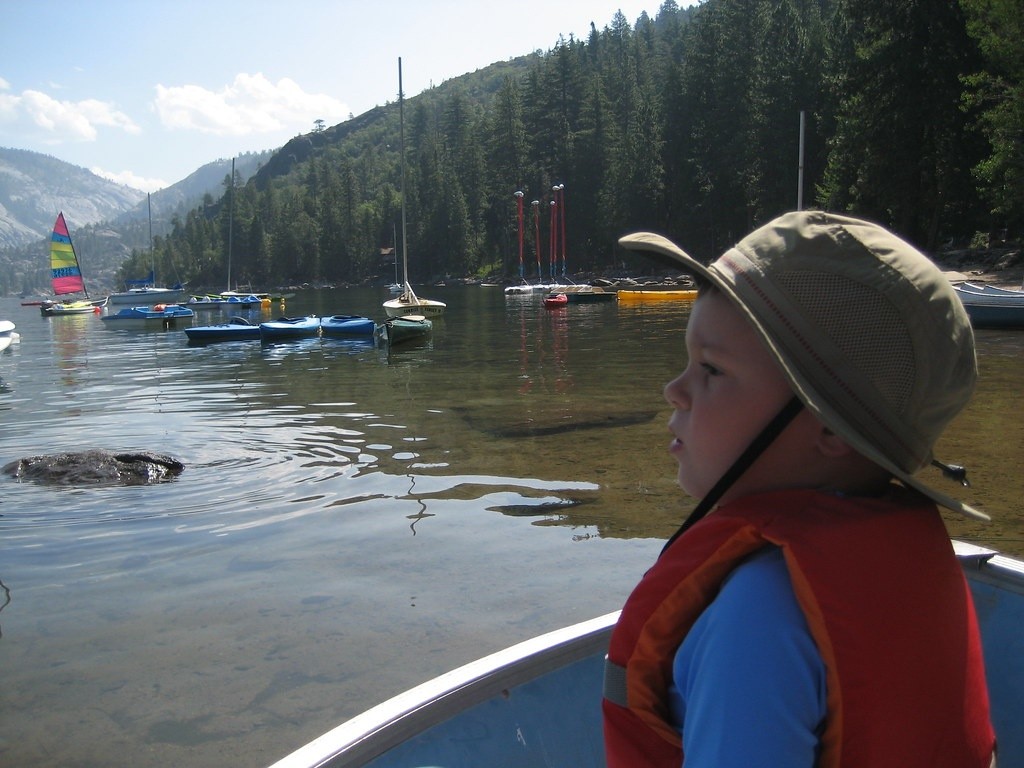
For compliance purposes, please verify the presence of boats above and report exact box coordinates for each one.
[182,311,377,346]
[21,299,42,306]
[950,280,1024,329]
[381,314,433,348]
[543,291,569,309]
[589,273,694,290]
[98,302,194,321]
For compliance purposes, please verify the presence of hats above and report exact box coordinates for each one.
[618,209,992,524]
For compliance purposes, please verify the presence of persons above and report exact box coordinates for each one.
[602,210,1000,768]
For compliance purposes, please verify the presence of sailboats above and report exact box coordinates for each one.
[175,158,275,312]
[109,191,191,306]
[491,182,590,296]
[382,56,448,320]
[38,208,108,318]
[388,224,405,293]
